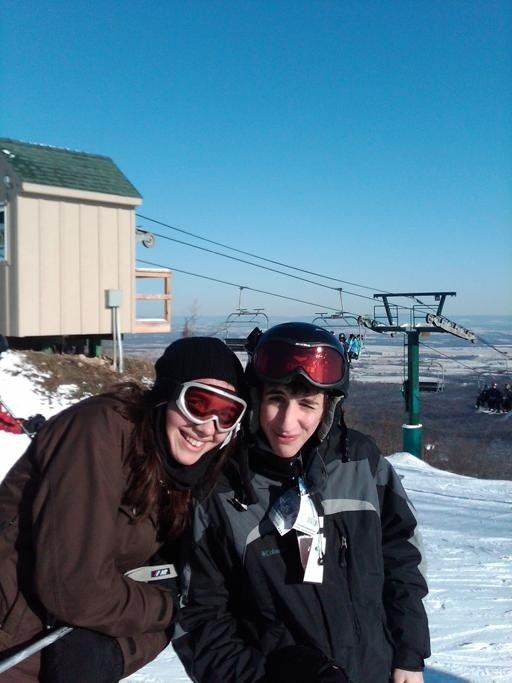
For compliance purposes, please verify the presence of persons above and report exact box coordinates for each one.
[167,321,434,683]
[0,336,245,683]
[330,330,364,363]
[473,383,512,413]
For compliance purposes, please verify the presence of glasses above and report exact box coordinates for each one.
[252,337,349,388]
[175,381,248,433]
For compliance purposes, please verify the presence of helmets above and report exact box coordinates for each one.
[244,322,350,439]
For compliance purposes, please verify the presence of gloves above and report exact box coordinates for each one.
[39,626,123,683]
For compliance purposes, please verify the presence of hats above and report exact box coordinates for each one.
[143,336,244,449]
